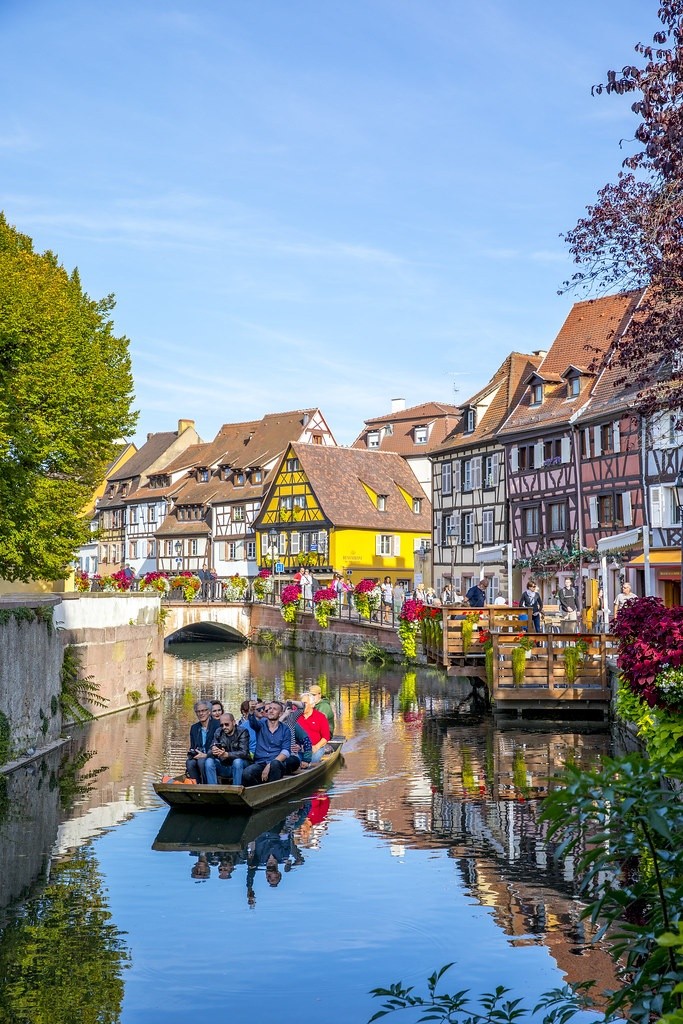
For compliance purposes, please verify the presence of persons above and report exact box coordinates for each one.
[494,592,506,605]
[191,792,330,910]
[196,564,215,602]
[238,685,334,769]
[614,583,638,621]
[210,700,224,720]
[204,713,250,785]
[124,563,134,578]
[597,588,604,612]
[293,563,450,623]
[186,700,221,784]
[519,581,543,648]
[559,577,578,620]
[242,700,291,786]
[463,580,489,632]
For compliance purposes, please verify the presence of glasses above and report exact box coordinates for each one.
[311,693,318,696]
[256,706,265,712]
[194,708,206,714]
[624,587,629,589]
[220,722,229,728]
[265,709,269,713]
[532,586,536,588]
[483,584,487,587]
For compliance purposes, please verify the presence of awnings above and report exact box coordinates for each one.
[476,545,507,561]
[596,526,642,553]
[629,551,681,564]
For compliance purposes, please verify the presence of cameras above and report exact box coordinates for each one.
[187,748,204,760]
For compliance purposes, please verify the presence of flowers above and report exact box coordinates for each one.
[397,599,444,658]
[395,672,424,730]
[430,739,528,806]
[478,629,492,700]
[280,586,302,621]
[313,588,337,628]
[139,571,203,602]
[354,580,376,619]
[221,568,273,602]
[654,662,683,715]
[563,633,593,687]
[462,611,483,659]
[75,569,135,592]
[511,632,537,689]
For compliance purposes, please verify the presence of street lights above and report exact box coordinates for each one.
[174,541,183,576]
[269,527,278,605]
[445,526,460,604]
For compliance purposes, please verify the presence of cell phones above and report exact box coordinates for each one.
[287,701,292,710]
[215,743,222,750]
[251,693,257,701]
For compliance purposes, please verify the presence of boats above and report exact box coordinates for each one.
[152,753,345,852]
[151,735,347,810]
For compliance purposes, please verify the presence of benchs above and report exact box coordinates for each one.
[294,735,347,774]
[538,605,560,634]
[187,773,294,784]
